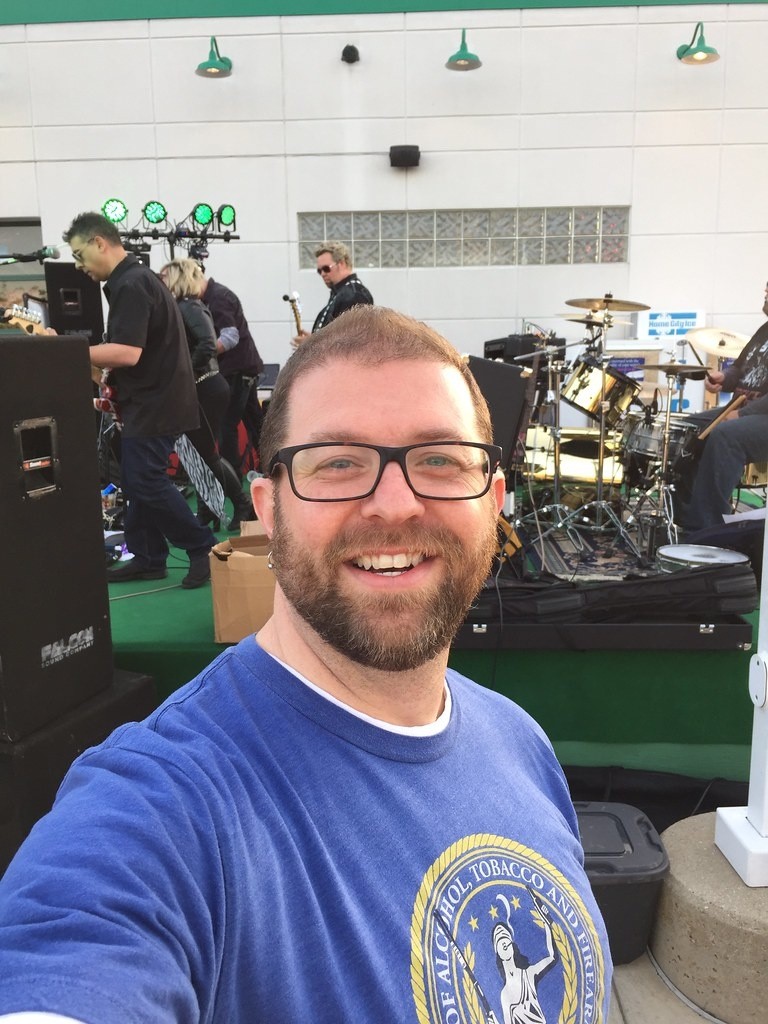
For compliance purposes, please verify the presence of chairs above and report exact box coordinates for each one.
[729,460,768,515]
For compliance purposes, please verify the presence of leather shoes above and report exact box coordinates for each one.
[182,536,223,588]
[108,556,167,582]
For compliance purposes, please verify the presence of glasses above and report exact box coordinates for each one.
[72,235,105,261]
[263,441,503,502]
[317,259,341,275]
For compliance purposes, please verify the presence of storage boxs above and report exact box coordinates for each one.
[209,520,279,644]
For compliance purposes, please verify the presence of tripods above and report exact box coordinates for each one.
[502,301,680,569]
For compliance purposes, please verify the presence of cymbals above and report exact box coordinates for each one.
[631,359,714,373]
[554,313,635,328]
[565,298,651,311]
[685,327,752,360]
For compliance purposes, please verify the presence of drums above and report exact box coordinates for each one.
[654,543,752,575]
[558,352,643,429]
[622,410,699,486]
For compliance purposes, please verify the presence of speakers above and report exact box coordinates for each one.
[0,332,114,744]
[44,261,105,348]
[467,355,532,476]
[391,145,419,167]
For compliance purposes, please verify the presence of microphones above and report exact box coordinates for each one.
[2,246,60,265]
[650,389,658,415]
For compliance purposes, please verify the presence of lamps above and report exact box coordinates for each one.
[101,198,238,233]
[390,145,421,167]
[677,22,722,65]
[194,36,232,78]
[443,28,482,72]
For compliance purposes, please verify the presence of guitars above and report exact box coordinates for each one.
[3,304,124,431]
[291,298,303,338]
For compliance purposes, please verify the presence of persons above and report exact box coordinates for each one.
[654,282,768,544]
[0,303,615,1024]
[198,276,265,488]
[288,241,374,349]
[41,213,221,588]
[160,258,258,531]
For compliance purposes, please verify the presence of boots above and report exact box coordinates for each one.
[194,492,221,532]
[217,457,258,531]
